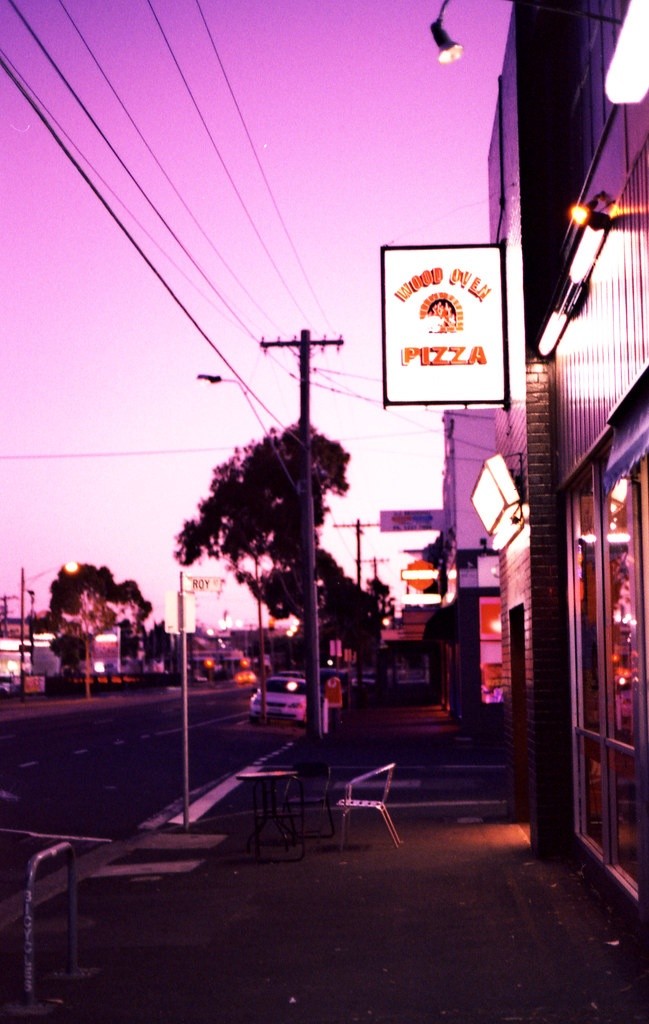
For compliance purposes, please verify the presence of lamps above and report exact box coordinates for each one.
[571,191,607,225]
[430,0,463,65]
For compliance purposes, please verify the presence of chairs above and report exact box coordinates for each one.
[274,761,335,844]
[337,763,402,851]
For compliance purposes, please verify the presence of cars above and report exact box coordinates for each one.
[249,676,307,725]
[276,666,378,708]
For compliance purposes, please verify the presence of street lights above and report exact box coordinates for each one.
[197,374,323,743]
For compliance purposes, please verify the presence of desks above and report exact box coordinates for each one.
[236,770,306,860]
